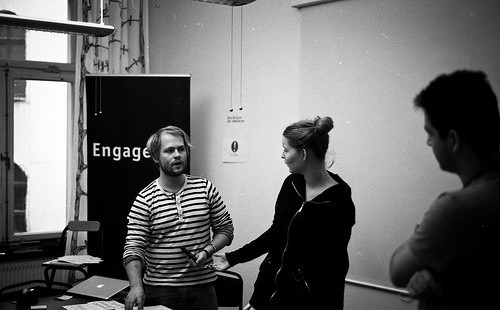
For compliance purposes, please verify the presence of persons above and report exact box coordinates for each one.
[202,114,356,310]
[388,68,496,310]
[122,126,234,310]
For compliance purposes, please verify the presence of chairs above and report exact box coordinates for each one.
[214,270,243,310]
[41,220,105,289]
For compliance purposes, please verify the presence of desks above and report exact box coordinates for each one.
[0,279,147,310]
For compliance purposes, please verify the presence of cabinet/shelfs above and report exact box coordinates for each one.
[86,72,192,291]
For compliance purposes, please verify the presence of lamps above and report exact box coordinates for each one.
[0,0,115,37]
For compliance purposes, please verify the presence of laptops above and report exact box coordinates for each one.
[66,275,130,300]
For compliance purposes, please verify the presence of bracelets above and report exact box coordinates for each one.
[202,242,217,258]
